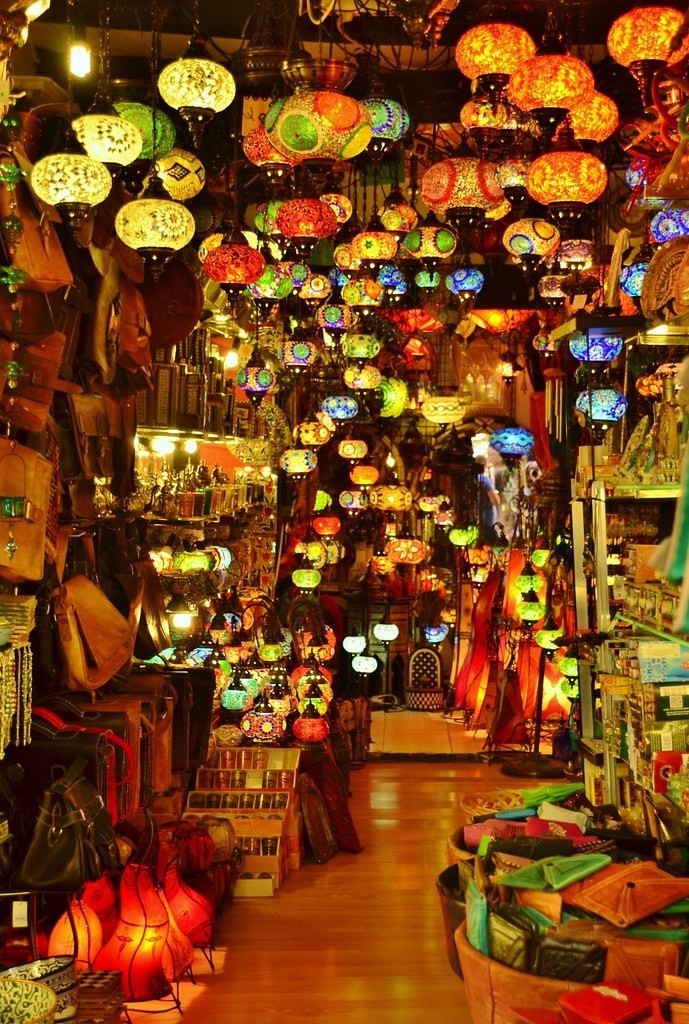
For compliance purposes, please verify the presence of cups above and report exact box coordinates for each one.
[181,484,253,518]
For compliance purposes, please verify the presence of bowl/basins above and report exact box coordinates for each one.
[0,955,77,992]
[54,980,80,1022]
[0,979,56,1024]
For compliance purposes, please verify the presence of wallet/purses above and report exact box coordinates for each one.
[461,784,689,1024]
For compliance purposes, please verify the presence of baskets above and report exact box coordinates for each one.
[459,786,522,822]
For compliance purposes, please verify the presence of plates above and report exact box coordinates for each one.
[640,236,689,326]
[619,415,650,477]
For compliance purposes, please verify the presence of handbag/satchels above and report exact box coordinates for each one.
[36,532,136,693]
[0,436,54,580]
[0,104,182,518]
[0,590,208,888]
[59,387,123,482]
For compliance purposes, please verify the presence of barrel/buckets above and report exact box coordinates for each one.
[447,827,475,865]
[435,858,475,981]
[453,918,593,1024]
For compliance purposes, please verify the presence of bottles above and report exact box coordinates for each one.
[255,478,264,501]
[247,478,254,502]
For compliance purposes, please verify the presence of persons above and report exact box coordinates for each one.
[476,444,515,540]
